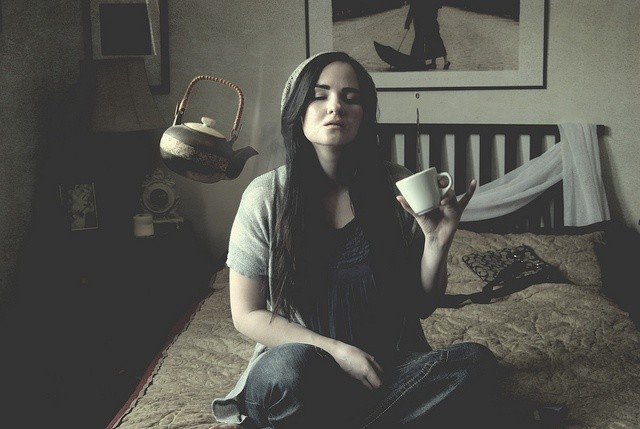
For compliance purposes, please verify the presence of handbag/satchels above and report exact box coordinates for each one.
[462,244,548,287]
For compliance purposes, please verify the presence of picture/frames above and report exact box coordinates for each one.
[304,0,548,91]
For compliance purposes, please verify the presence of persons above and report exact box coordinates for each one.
[211,50,498,426]
[404,1,451,70]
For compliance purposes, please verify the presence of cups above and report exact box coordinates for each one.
[393,166,453,217]
[133,214,154,238]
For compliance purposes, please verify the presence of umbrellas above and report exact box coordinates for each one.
[374,26,409,71]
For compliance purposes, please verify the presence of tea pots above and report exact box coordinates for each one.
[159,76,259,185]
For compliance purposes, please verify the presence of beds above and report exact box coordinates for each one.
[106,123,636,429]
